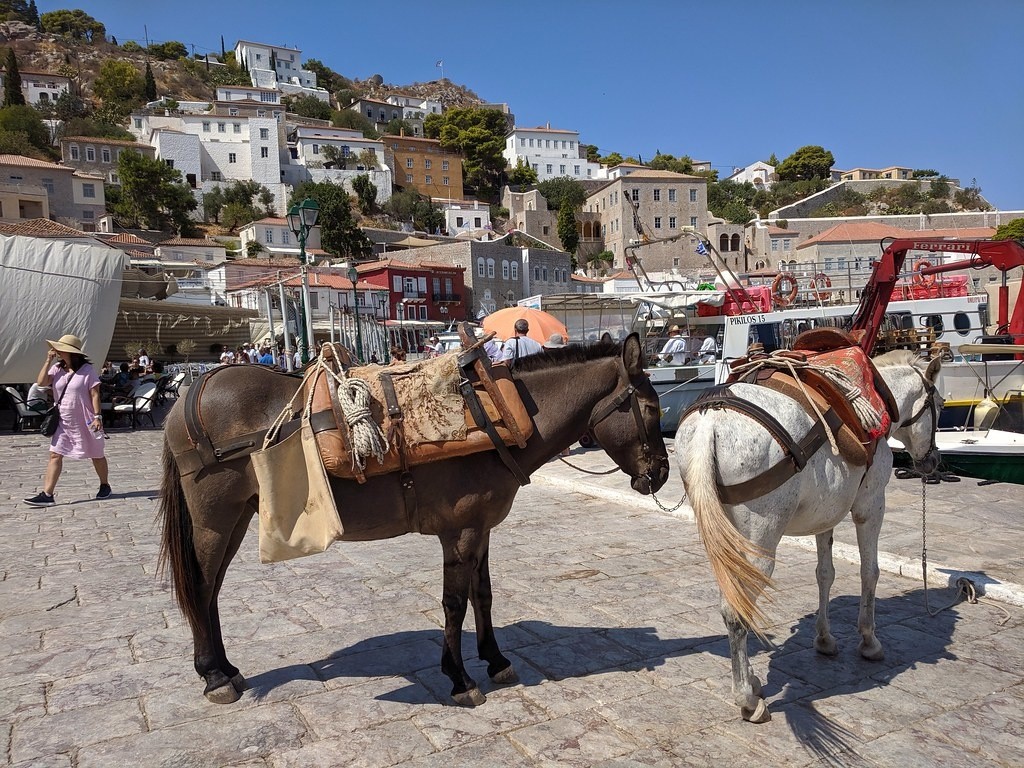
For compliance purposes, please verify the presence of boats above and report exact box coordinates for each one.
[624,189,1024,486]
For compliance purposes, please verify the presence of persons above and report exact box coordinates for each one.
[502,319,545,360]
[428,337,445,359]
[220,329,325,372]
[99,349,165,400]
[22,334,113,506]
[697,336,716,365]
[655,325,687,367]
[27,383,52,427]
[371,351,380,364]
[545,332,577,454]
[391,344,406,361]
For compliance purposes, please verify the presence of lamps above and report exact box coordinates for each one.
[681,226,695,233]
[629,238,645,245]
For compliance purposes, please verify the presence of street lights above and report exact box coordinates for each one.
[440,307,448,324]
[377,289,390,365]
[348,266,365,364]
[396,302,404,329]
[285,195,321,375]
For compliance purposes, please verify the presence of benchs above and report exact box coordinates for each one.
[943,398,1011,407]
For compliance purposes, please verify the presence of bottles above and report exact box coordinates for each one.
[150,360,153,366]
[87,421,103,440]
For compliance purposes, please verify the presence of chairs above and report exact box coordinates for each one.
[6,386,50,431]
[99,366,186,429]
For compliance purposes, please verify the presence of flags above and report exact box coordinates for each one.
[461,222,469,228]
[436,59,443,67]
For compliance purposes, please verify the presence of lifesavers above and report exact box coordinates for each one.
[771,274,799,307]
[810,274,833,302]
[913,260,938,288]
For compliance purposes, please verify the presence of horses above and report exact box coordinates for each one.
[672,338,944,724]
[160,331,669,707]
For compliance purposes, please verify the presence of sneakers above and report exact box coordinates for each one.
[96,484,112,499]
[23,492,55,506]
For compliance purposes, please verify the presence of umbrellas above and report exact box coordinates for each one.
[482,305,569,346]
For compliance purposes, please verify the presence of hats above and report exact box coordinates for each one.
[666,325,681,336]
[243,343,249,346]
[46,334,88,358]
[544,334,568,348]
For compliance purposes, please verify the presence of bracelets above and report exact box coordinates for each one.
[94,414,102,419]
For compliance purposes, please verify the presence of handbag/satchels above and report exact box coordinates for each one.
[40,406,60,437]
